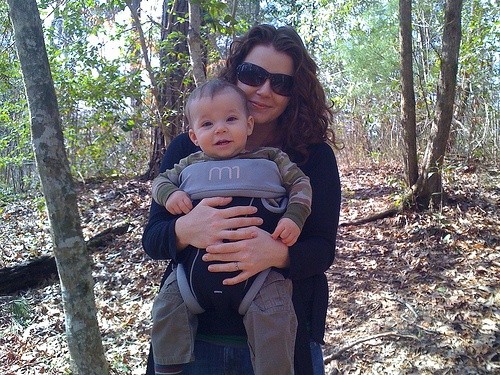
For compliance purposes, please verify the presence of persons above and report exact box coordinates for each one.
[150,80,312,375]
[142,24,341,375]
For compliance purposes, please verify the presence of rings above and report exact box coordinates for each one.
[235,262,239,271]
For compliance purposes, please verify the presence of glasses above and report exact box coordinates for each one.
[236,61,294,97]
